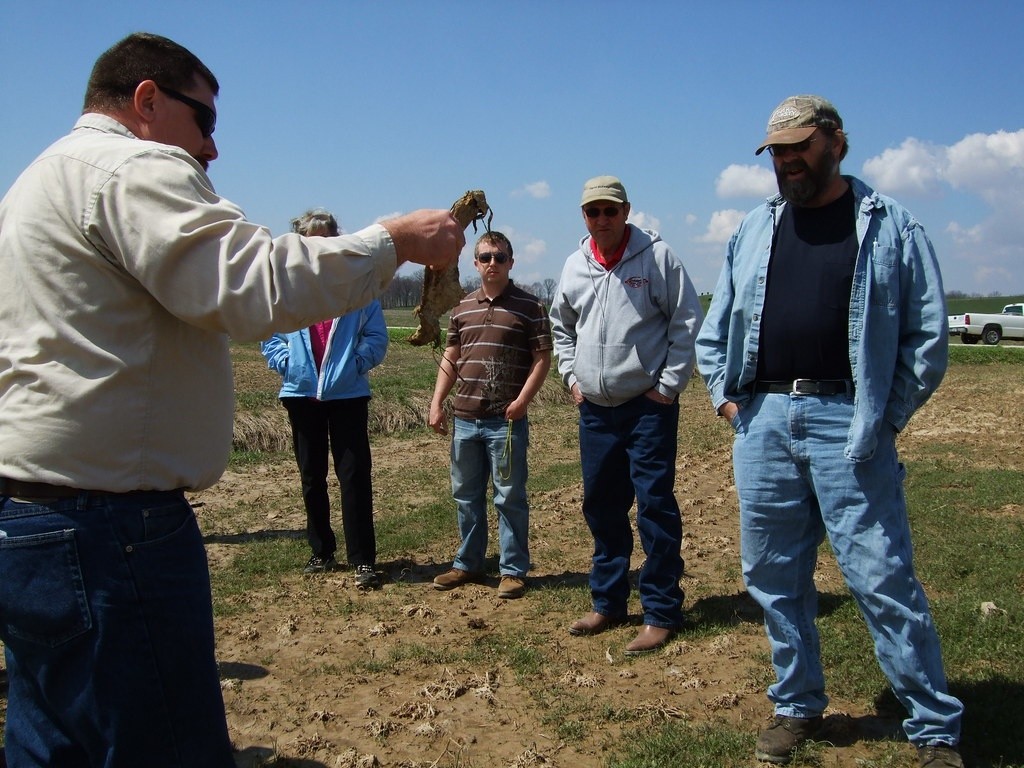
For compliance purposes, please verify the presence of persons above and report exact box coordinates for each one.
[0,32,466,768]
[258,209,390,588]
[548,175,705,653]
[427,231,553,599]
[695,94,966,767]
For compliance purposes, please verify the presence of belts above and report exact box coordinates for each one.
[0,477,111,500]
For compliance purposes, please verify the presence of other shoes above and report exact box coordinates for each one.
[914,743,964,768]
[355,563,380,590]
[498,574,525,598]
[433,568,478,590]
[755,715,824,764]
[304,555,338,573]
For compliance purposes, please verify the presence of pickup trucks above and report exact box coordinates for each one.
[947,303,1024,345]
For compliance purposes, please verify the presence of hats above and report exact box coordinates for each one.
[756,95,843,155]
[579,176,627,206]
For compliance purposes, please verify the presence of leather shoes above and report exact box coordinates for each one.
[748,378,855,395]
[624,624,680,655]
[568,611,627,635]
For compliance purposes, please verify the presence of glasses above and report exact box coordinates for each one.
[583,207,624,218]
[766,134,827,156]
[159,85,217,139]
[476,252,511,263]
